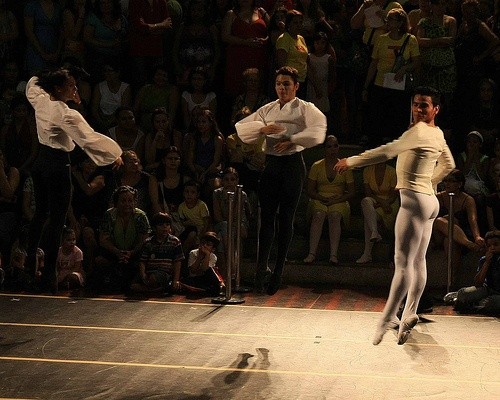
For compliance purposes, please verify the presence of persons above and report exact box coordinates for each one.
[180,232,226,294]
[356,161,400,263]
[333,87,454,345]
[26,69,124,292]
[236,66,326,295]
[94,185,151,290]
[443,230,500,312]
[303,135,354,263]
[349,0,500,290]
[0,0,336,287]
[130,215,186,296]
[55,229,87,289]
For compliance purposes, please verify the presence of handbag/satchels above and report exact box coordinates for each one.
[390,35,416,93]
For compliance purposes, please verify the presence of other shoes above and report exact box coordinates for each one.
[303,252,317,265]
[329,255,338,264]
[369,231,382,242]
[355,255,372,264]
